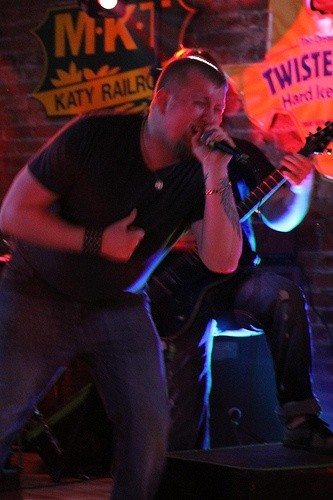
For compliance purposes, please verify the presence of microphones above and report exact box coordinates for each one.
[200,131,251,165]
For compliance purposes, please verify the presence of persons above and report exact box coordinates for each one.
[147,48,333,454]
[0,56,242,500]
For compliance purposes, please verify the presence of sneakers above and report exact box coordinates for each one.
[285,416,333,454]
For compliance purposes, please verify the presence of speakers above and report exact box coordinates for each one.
[156,443,333,500]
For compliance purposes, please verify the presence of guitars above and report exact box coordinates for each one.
[145,118,333,341]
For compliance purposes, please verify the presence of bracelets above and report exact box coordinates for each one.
[83,227,103,258]
[205,182,231,196]
